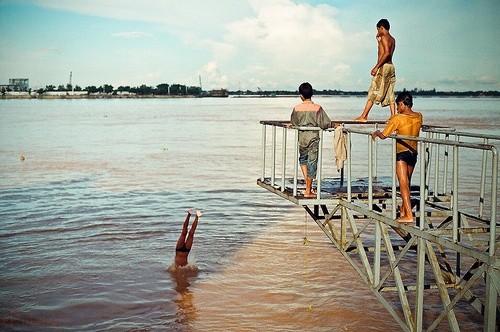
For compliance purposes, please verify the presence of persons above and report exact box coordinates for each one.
[372,93,423,222]
[354,19,396,122]
[174,208,202,267]
[288,82,340,197]
